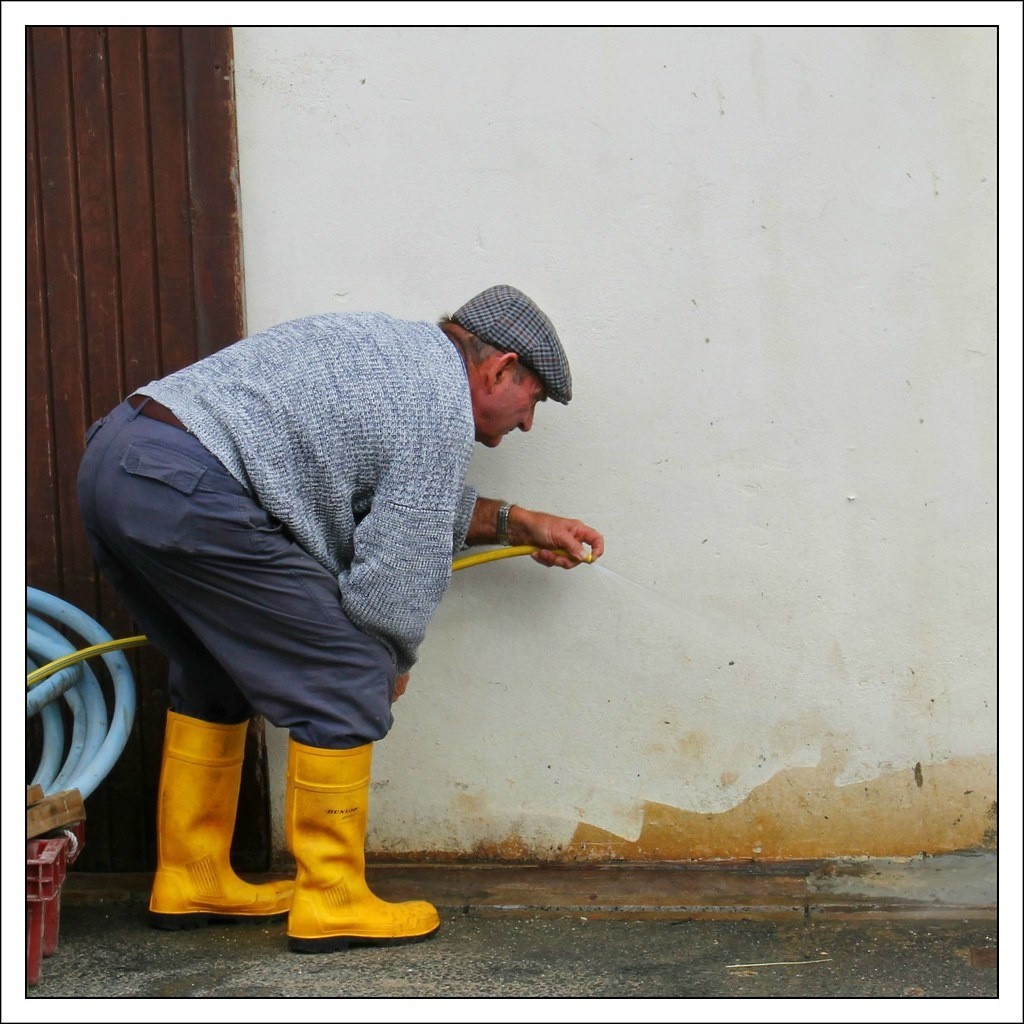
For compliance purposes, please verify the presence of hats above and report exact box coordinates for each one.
[451,285,573,406]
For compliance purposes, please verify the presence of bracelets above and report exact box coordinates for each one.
[493,500,518,545]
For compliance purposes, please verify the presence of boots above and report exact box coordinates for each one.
[147,708,295,929]
[284,734,441,954]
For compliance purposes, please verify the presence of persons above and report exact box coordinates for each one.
[75,284,605,954]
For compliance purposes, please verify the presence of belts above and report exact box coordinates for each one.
[127,394,188,430]
